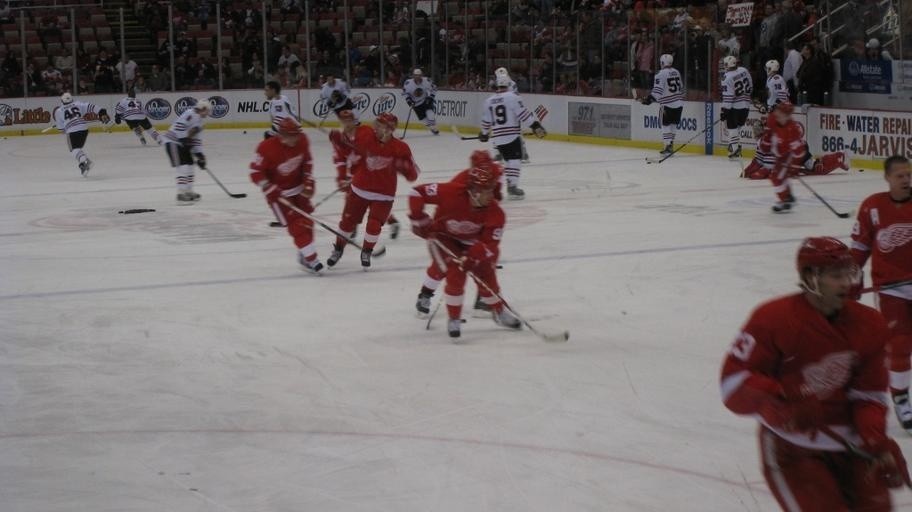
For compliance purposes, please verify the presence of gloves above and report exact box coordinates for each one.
[336,176,352,193]
[298,181,314,198]
[406,97,415,107]
[425,96,434,106]
[197,159,205,167]
[478,133,488,143]
[641,95,656,105]
[529,121,547,138]
[869,438,911,489]
[408,213,435,239]
[98,108,110,124]
[392,155,413,178]
[115,113,121,124]
[262,182,282,204]
[763,395,824,434]
[453,243,485,272]
[720,108,727,122]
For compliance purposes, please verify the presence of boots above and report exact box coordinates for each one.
[728,144,740,157]
[660,145,673,154]
[892,393,912,429]
[773,196,798,211]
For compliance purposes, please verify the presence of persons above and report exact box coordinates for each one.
[850,154,910,431]
[721,235,910,511]
[249,62,546,335]
[373,1,654,99]
[643,0,902,214]
[58,87,213,207]
[133,0,373,90]
[0,0,140,98]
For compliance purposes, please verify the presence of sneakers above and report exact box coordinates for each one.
[508,186,524,196]
[188,192,200,199]
[78,163,89,174]
[301,221,400,271]
[177,193,192,201]
[416,291,521,337]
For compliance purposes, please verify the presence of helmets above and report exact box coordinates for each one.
[495,67,508,78]
[61,92,74,104]
[465,149,505,195]
[796,236,855,304]
[660,54,674,67]
[195,98,210,111]
[278,117,302,135]
[413,68,422,75]
[496,77,510,87]
[340,109,355,120]
[765,61,779,74]
[377,112,398,129]
[724,57,737,69]
[777,100,793,113]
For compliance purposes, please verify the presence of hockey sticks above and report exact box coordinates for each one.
[797,175,855,217]
[818,424,912,489]
[270,180,349,227]
[280,197,385,256]
[203,166,247,198]
[435,238,569,343]
[451,127,535,140]
[399,105,415,140]
[646,117,722,164]
